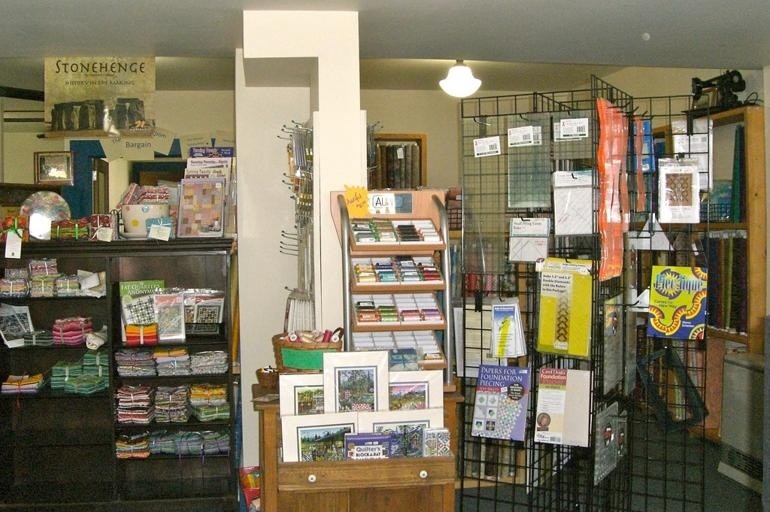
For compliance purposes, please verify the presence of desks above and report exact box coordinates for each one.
[251,383,466,512]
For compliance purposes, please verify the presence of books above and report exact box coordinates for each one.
[604,293,623,395]
[624,306,637,396]
[534,367,593,447]
[187,158,233,171]
[618,411,630,462]
[178,176,225,237]
[342,432,391,461]
[424,427,452,457]
[185,168,229,195]
[595,401,619,485]
[472,366,530,442]
[190,146,233,158]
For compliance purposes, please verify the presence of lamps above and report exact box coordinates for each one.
[437,57,484,103]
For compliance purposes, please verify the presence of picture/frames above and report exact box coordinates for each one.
[280,412,355,461]
[34,152,75,187]
[322,350,389,412]
[356,409,448,458]
[279,374,323,416]
[126,157,191,190]
[388,370,444,408]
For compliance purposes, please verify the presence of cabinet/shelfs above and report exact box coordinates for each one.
[626,104,769,449]
[328,189,459,395]
[0,238,243,512]
[367,131,430,193]
[718,349,768,498]
[452,73,712,512]
